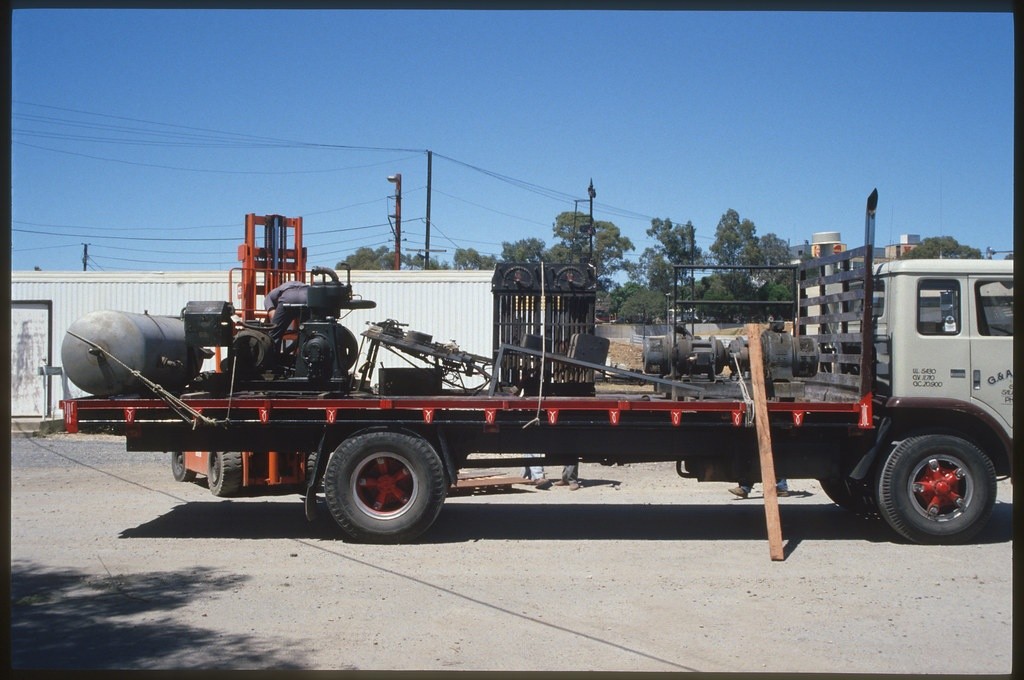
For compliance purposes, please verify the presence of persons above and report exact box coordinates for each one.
[522,454,579,490]
[264,281,310,348]
[728,479,788,498]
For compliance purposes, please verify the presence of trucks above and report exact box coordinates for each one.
[58,178,1013,547]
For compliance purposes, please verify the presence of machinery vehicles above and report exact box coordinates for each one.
[170,214,314,497]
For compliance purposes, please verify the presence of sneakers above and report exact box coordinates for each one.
[762,491,790,497]
[728,487,747,498]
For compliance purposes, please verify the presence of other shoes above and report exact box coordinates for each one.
[570,482,579,490]
[555,481,569,486]
